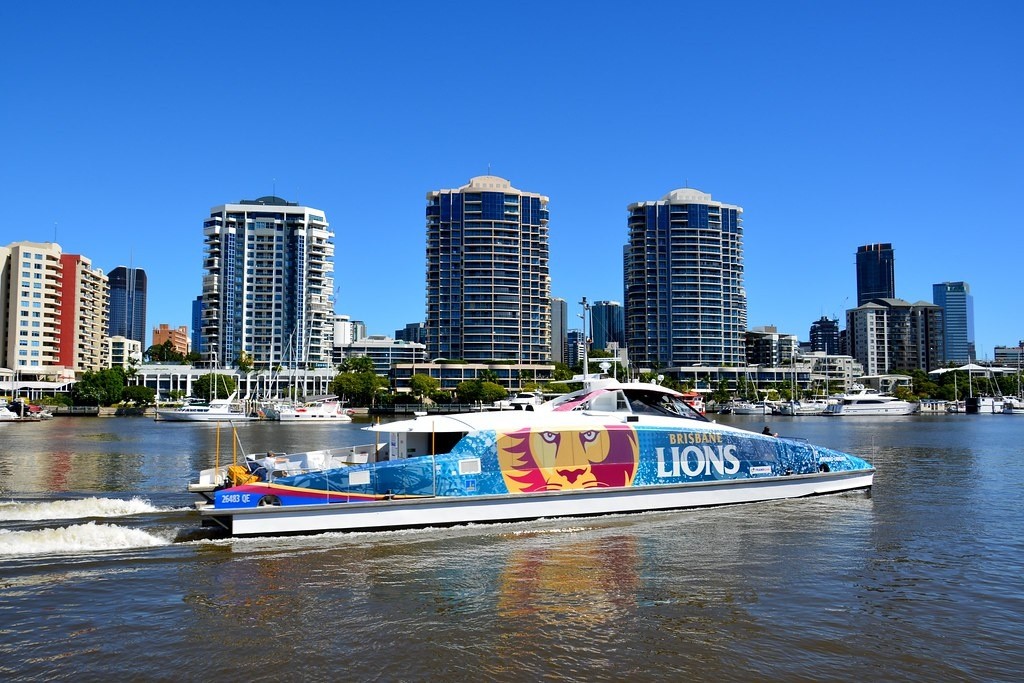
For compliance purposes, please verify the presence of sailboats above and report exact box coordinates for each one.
[273,312,352,423]
[1001,351,1024,413]
[770,342,916,418]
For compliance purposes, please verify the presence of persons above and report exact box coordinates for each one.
[762,426,778,436]
[265,451,288,478]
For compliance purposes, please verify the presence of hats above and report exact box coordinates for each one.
[267,450,274,456]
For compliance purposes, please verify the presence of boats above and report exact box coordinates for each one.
[677,392,706,418]
[180,297,879,536]
[718,361,774,416]
[154,352,261,422]
